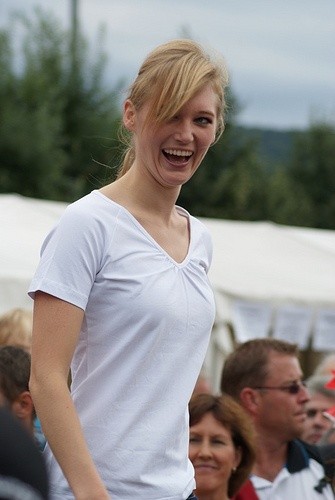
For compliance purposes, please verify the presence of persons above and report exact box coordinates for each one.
[26,39,229,500]
[0,307,335,500]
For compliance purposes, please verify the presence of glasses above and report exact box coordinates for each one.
[256,384,308,395]
[307,408,317,418]
